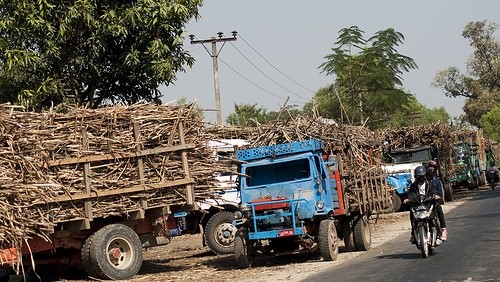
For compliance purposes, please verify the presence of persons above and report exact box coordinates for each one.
[474,159,500,186]
[404,161,447,244]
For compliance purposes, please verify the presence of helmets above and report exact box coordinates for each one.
[425,159,437,170]
[414,166,427,177]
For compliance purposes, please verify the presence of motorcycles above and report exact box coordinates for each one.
[403,188,441,258]
[487,171,498,190]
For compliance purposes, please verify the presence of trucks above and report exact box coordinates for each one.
[151,122,494,261]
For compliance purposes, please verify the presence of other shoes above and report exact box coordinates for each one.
[440,227,449,242]
[410,235,416,244]
[436,238,442,245]
